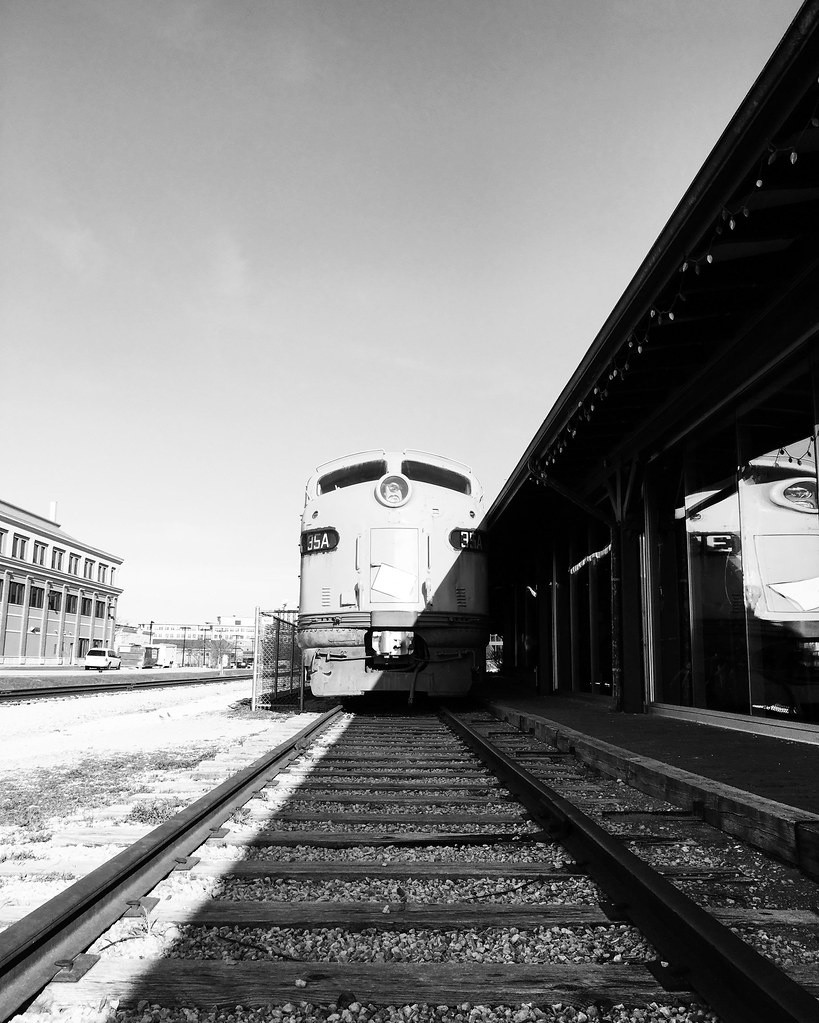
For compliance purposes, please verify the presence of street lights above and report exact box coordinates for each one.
[200,629,213,665]
[180,627,191,666]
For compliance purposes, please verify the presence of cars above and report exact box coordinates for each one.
[83,646,122,669]
[231,662,250,669]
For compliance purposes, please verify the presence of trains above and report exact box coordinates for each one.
[294,445,488,709]
[502,458,819,717]
